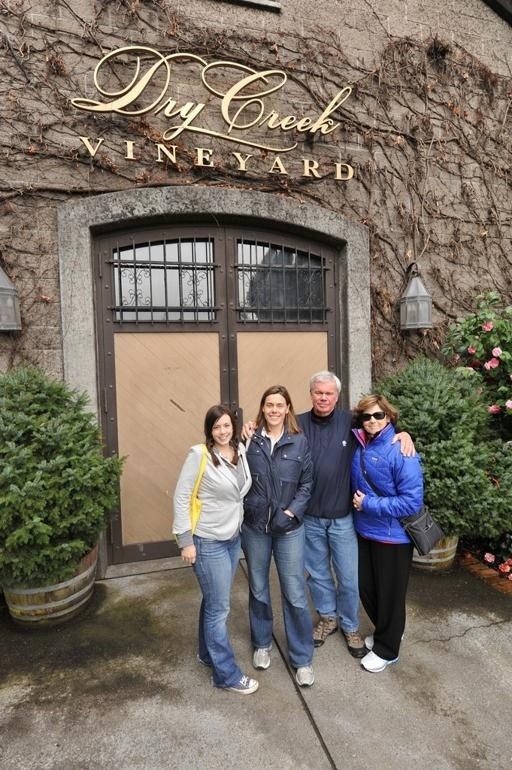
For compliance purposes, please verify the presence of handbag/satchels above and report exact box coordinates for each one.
[173,444,206,546]
[360,445,444,556]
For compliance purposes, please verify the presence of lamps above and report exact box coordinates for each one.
[0,268,22,331]
[400,266,432,330]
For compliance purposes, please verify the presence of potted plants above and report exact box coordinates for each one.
[1,370,121,624]
[365,362,512,566]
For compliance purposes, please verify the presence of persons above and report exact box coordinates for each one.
[241,385,315,689]
[171,404,262,696]
[242,373,414,657]
[351,394,421,673]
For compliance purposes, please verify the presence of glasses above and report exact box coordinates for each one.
[361,411,387,422]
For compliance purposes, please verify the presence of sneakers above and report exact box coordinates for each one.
[253,645,273,669]
[225,675,259,694]
[196,651,213,665]
[344,632,367,658]
[360,650,400,673]
[364,635,404,650]
[296,666,314,687]
[313,618,338,647]
